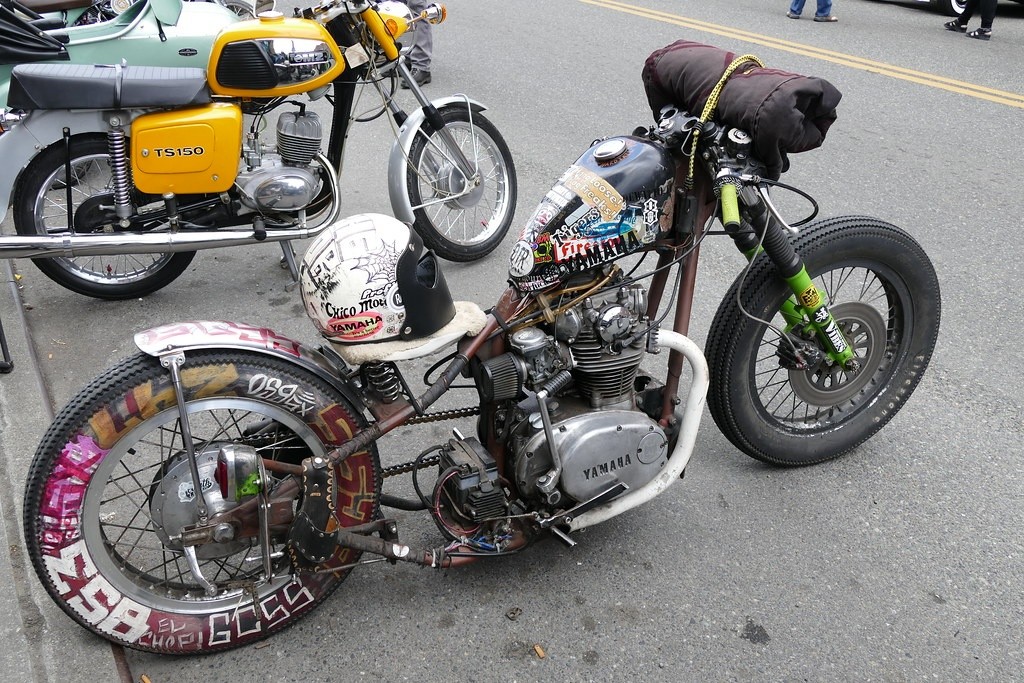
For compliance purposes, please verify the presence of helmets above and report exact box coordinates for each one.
[299,213,458,346]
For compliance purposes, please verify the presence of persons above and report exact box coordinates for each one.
[786,0,839,22]
[944,0,998,40]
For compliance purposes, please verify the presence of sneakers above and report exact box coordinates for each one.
[380,63,410,77]
[403,69,433,86]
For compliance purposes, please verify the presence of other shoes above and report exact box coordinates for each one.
[814,14,838,22]
[786,11,799,19]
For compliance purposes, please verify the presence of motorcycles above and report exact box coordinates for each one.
[0,0,518,300]
[23,104,941,657]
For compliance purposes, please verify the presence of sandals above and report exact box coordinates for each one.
[965,28,991,40]
[944,19,967,33]
